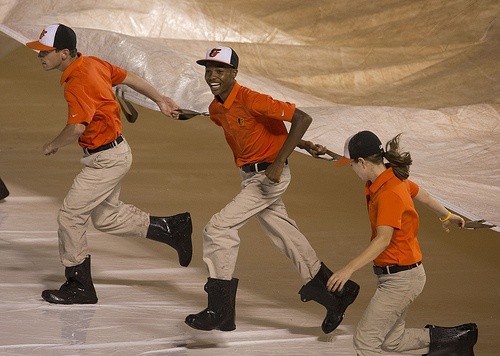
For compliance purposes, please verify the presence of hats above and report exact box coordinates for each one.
[27,24,76,50]
[333,131,384,166]
[196,45,239,70]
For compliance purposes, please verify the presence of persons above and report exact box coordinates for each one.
[184,45,361,334]
[327,130,479,356]
[26,24,193,305]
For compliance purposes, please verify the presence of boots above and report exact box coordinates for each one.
[41,255,99,305]
[297,261,360,334]
[146,212,193,268]
[184,277,239,331]
[426,323,478,356]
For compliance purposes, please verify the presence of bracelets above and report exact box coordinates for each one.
[439,211,452,222]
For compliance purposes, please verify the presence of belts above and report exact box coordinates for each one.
[240,158,289,172]
[83,135,124,155]
[373,262,420,276]
